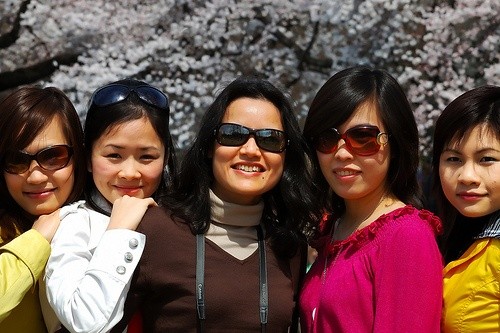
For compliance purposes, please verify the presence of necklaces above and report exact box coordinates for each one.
[324,193,391,283]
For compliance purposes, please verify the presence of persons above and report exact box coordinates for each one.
[297,64,445,333]
[0,86,89,333]
[38,80,179,333]
[432,86,500,333]
[54,74,320,333]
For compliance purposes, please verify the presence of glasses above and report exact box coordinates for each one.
[88,84,170,109]
[312,124,391,156]
[0,144,74,174]
[212,123,290,153]
